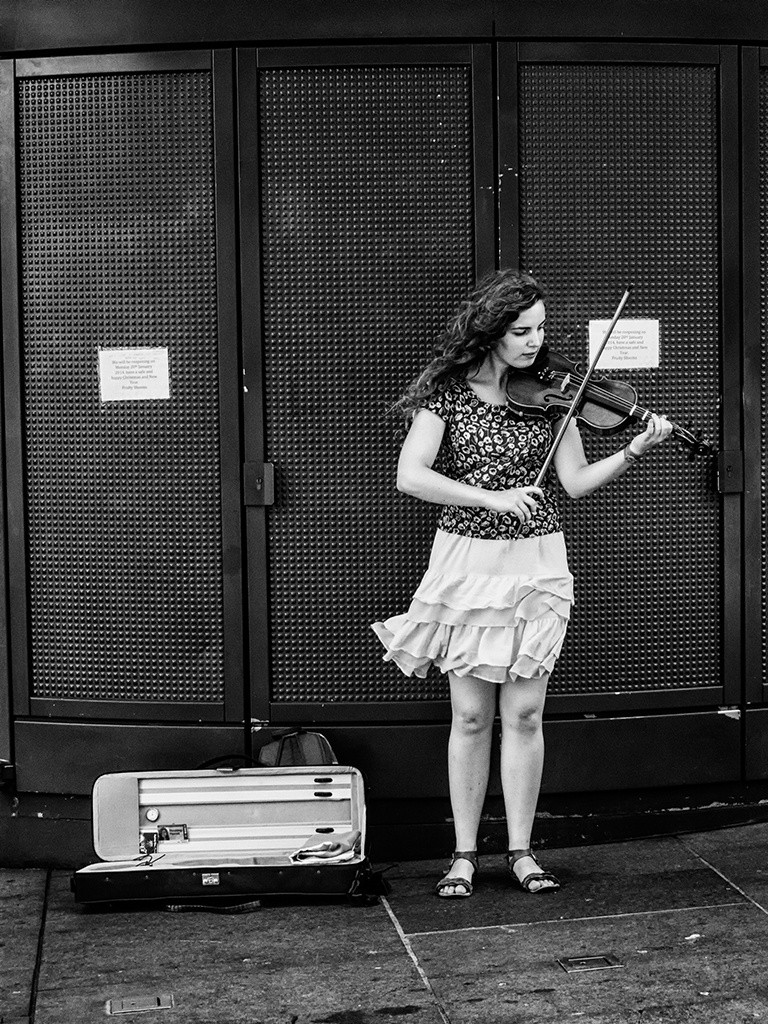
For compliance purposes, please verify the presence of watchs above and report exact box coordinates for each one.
[624,444,640,465]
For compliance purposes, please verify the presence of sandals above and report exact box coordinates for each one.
[505,848,562,894]
[434,849,479,897]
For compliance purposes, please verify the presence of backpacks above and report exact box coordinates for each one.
[256,724,339,767]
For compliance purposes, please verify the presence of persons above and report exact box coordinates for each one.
[395,268,673,897]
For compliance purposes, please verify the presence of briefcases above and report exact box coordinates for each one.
[69,755,380,915]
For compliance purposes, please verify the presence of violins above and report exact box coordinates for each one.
[504,351,715,456]
